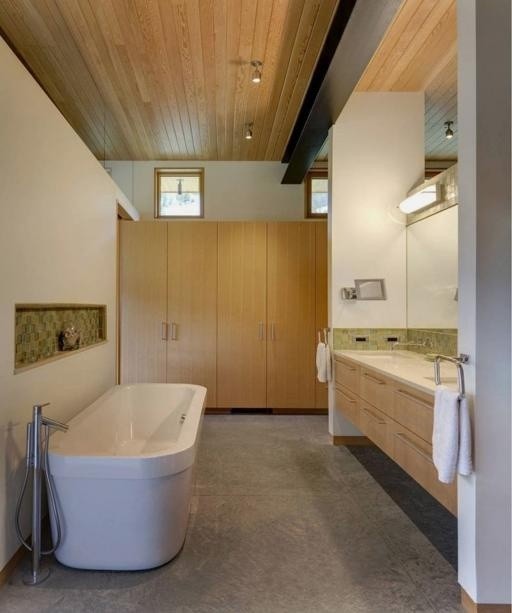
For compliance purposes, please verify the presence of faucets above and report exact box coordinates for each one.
[391,339,428,351]
[29,401,69,587]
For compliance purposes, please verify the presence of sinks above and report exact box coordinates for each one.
[357,350,405,357]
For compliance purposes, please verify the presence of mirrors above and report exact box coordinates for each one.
[341,277,386,300]
[406,202,458,364]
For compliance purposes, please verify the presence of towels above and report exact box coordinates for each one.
[432,385,473,483]
[315,342,331,383]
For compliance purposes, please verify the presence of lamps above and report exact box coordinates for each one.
[443,120,454,139]
[244,121,253,140]
[250,58,263,82]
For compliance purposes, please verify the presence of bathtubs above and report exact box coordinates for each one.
[41,382,207,479]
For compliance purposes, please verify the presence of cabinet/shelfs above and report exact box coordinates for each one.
[332,353,458,517]
[218,221,315,408]
[120,221,217,408]
[316,221,328,408]
